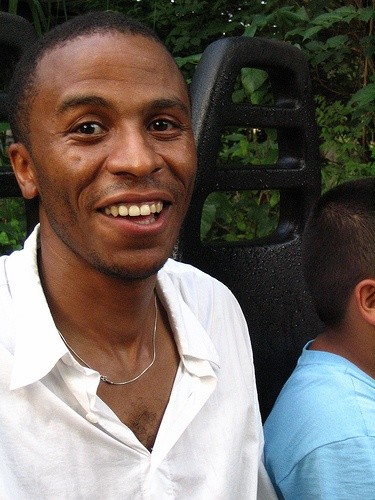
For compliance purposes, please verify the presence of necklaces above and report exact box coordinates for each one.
[56,287,159,385]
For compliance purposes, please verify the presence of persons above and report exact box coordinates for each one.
[0,12,278,500]
[263,177,374,499]
